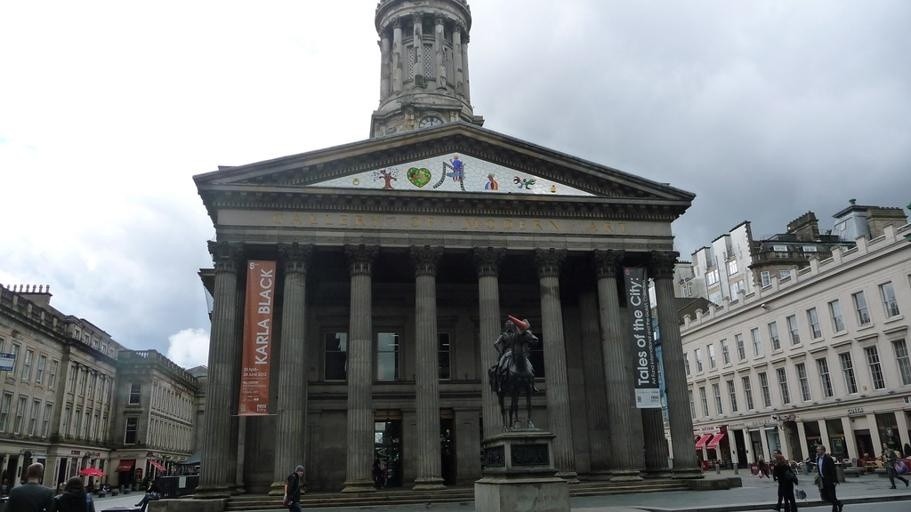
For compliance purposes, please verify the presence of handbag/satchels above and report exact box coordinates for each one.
[795,488,807,500]
[758,470,763,478]
[894,461,907,474]
[282,496,294,505]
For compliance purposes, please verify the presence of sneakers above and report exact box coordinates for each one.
[889,486,897,489]
[760,477,771,479]
[773,507,780,511]
[135,503,141,507]
[906,480,909,487]
[831,502,843,512]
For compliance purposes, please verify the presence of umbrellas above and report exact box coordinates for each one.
[76,468,105,487]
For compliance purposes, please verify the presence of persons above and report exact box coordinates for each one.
[774,455,798,512]
[697,452,720,474]
[374,458,386,489]
[159,468,199,499]
[881,441,909,489]
[731,450,739,474]
[770,449,788,511]
[51,476,94,512]
[88,477,111,495]
[286,465,306,512]
[3,462,54,512]
[859,452,876,464]
[133,480,159,512]
[815,445,843,511]
[494,319,540,393]
[757,452,844,479]
[386,458,402,488]
[878,443,911,466]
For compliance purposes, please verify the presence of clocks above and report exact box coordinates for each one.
[416,115,446,131]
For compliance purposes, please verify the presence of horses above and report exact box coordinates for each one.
[495,326,539,433]
